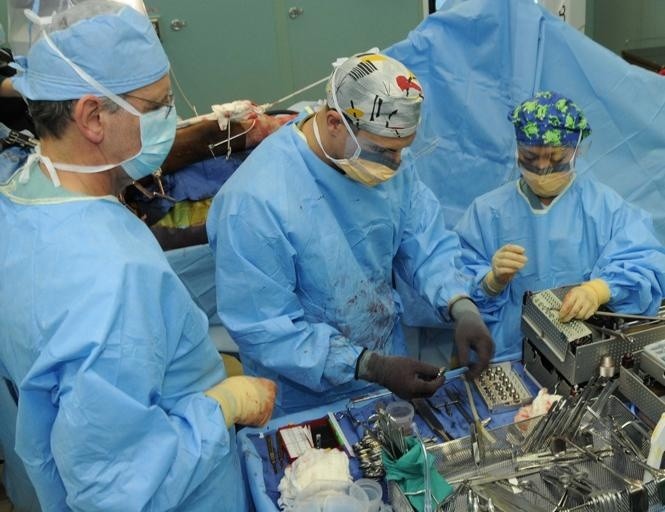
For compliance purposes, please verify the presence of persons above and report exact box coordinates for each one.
[206,51,496,417]
[1,6,277,512]
[451,90,664,358]
[125,113,298,251]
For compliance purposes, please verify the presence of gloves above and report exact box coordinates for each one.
[485,242,526,293]
[557,276,612,324]
[352,348,447,402]
[208,375,277,428]
[449,295,495,380]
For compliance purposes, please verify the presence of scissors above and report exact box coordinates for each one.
[334,398,436,478]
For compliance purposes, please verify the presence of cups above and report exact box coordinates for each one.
[349,478,383,512]
[385,401,414,436]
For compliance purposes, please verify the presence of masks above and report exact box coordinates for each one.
[87,95,177,182]
[514,151,576,198]
[327,122,402,186]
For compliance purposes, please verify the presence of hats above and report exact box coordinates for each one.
[507,90,592,149]
[328,50,424,137]
[12,0,170,103]
